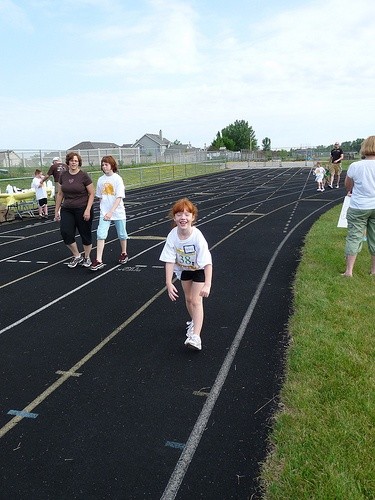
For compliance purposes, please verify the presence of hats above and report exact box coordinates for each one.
[52,157,62,163]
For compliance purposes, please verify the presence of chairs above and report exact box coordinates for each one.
[8,199,36,217]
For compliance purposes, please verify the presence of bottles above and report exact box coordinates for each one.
[47,180,52,187]
[6,184,13,193]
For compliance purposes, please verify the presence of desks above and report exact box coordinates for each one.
[0,187,54,221]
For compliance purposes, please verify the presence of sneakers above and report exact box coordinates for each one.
[183,336,202,355]
[89,260,103,271]
[186,320,194,337]
[68,256,84,268]
[82,258,92,267]
[119,253,128,264]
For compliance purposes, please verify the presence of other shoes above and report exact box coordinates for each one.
[328,185,333,189]
[317,188,321,191]
[38,216,42,220]
[321,189,325,191]
[335,185,339,189]
[45,215,48,219]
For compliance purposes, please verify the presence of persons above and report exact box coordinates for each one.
[323,161,331,188]
[338,135,375,277]
[313,162,327,192]
[31,168,49,217]
[39,159,69,221]
[329,141,344,189]
[54,152,95,268]
[89,155,129,271]
[158,198,213,352]
